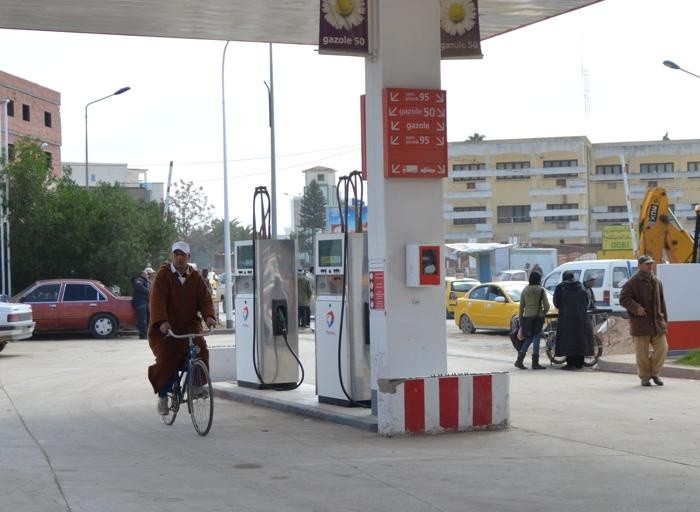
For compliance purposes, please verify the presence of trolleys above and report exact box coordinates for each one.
[517,307,615,365]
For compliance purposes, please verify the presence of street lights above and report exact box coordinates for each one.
[0,142,49,300]
[283,192,302,230]
[662,60,699,79]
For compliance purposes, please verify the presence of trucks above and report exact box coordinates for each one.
[498,269,527,282]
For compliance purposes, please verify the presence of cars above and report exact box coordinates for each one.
[0,294,10,302]
[5,279,140,339]
[0,301,37,351]
[209,271,234,313]
[452,280,562,334]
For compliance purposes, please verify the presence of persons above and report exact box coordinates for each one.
[523,262,543,278]
[618,255,670,386]
[308,266,314,328]
[147,241,216,416]
[298,266,311,329]
[132,267,156,339]
[200,268,213,295]
[304,270,312,280]
[514,271,550,369]
[581,272,597,304]
[553,270,589,370]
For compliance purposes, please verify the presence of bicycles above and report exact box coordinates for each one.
[158,321,219,438]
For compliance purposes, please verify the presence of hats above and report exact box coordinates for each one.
[637,255,657,265]
[172,241,192,255]
[145,267,156,275]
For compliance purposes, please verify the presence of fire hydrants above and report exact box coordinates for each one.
[84,86,132,190]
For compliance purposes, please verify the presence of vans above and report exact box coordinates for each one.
[540,259,641,318]
[444,275,483,318]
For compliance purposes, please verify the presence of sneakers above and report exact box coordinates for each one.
[652,372,665,387]
[641,381,651,387]
[191,387,208,399]
[157,394,170,416]
[561,362,578,371]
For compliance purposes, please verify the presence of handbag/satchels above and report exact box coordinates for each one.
[522,318,543,336]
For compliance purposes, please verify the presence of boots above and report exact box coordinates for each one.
[531,353,546,369]
[514,351,528,370]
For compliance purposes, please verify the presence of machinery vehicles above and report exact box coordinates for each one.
[637,185,698,263]
